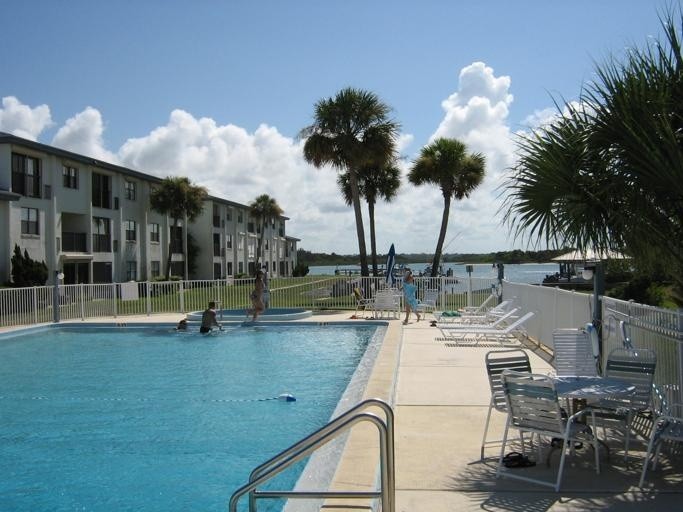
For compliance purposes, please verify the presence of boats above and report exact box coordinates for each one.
[532,247,634,290]
[412,263,458,284]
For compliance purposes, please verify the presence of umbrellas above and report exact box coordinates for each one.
[383,243,397,318]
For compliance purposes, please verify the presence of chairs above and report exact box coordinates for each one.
[582,348,657,462]
[416,289,438,320]
[432,294,535,348]
[639,404,683,489]
[353,286,402,320]
[553,328,597,379]
[480,349,535,460]
[494,369,600,493]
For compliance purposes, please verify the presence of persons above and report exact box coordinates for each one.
[173,320,190,331]
[400,273,421,326]
[199,301,223,333]
[248,270,266,322]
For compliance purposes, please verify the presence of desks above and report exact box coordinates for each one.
[532,377,637,462]
